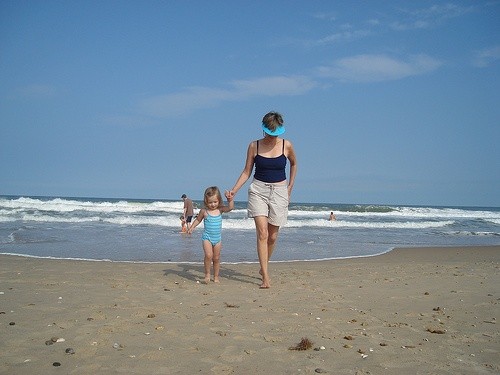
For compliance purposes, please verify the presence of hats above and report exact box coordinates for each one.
[181,194,187,198]
[261,123,285,136]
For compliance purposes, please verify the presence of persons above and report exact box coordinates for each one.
[188,186,234,283]
[194,214,198,219]
[329,212,336,221]
[181,194,194,230]
[225,112,296,288]
[180,216,187,232]
